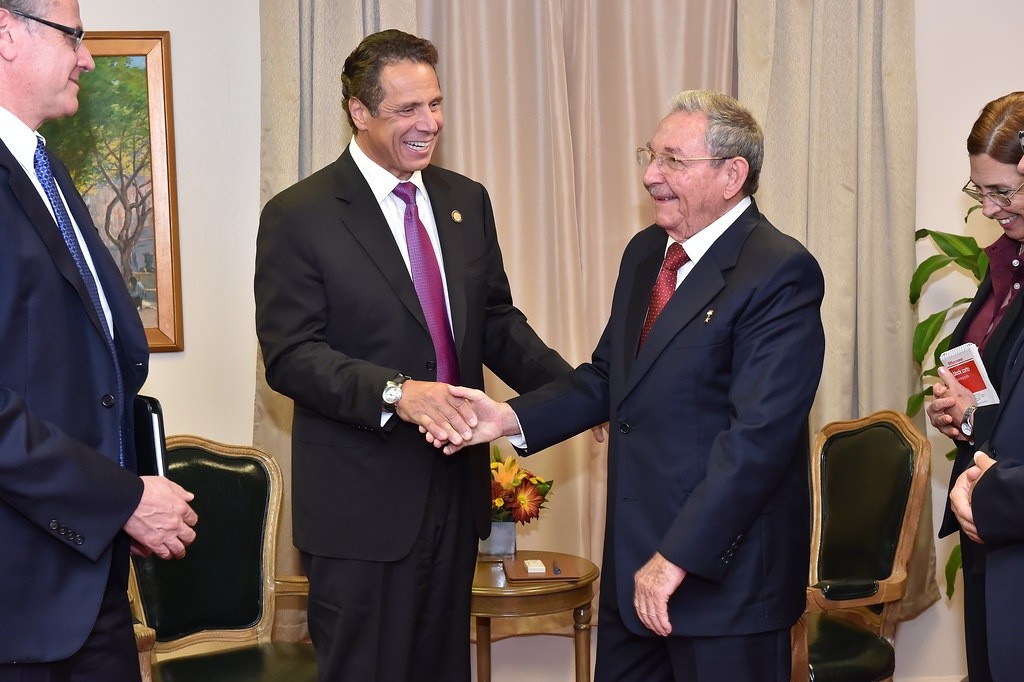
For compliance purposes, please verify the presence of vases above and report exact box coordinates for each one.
[478,522,518,560]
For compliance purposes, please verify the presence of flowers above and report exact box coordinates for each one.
[489,445,554,527]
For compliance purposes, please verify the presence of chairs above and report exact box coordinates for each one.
[792,408,930,682]
[123,434,311,681]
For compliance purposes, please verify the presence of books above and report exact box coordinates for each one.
[940,343,1000,406]
[137,391,169,477]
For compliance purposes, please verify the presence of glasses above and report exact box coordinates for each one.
[11,8,86,52]
[634,148,734,174]
[961,178,1024,207]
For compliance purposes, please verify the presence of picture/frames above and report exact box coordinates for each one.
[39,31,184,354]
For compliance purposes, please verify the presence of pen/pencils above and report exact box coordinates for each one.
[552,558,561,575]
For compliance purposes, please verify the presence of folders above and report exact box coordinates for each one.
[133,392,169,478]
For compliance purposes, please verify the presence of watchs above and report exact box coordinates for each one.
[382,375,412,413]
[960,406,978,440]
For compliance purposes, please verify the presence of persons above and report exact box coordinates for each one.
[253,28,610,682]
[927,92,1024,682]
[419,89,825,682]
[0,0,197,682]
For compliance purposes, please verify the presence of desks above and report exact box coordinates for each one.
[469,550,602,682]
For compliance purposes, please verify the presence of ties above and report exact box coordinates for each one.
[393,182,461,387]
[637,242,691,348]
[34,136,124,468]
